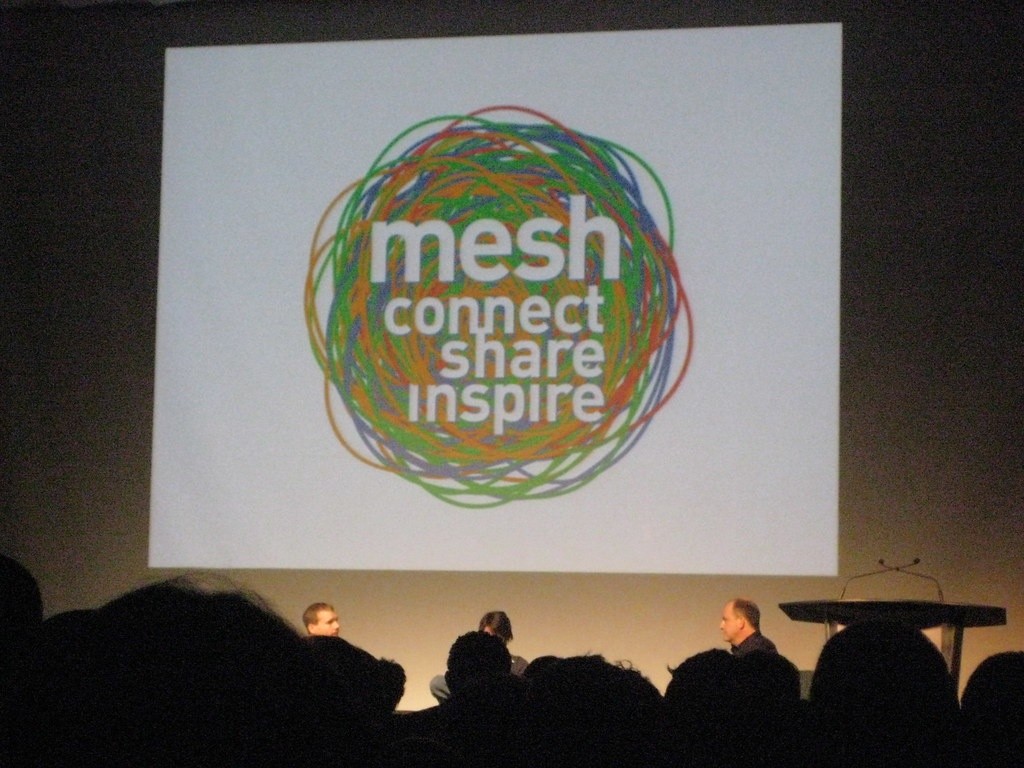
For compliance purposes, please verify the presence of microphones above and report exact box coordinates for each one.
[839,558,944,602]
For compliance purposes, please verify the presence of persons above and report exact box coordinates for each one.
[301,603,339,637]
[720,599,779,656]
[0,553,1024,768]
[479,611,528,678]
